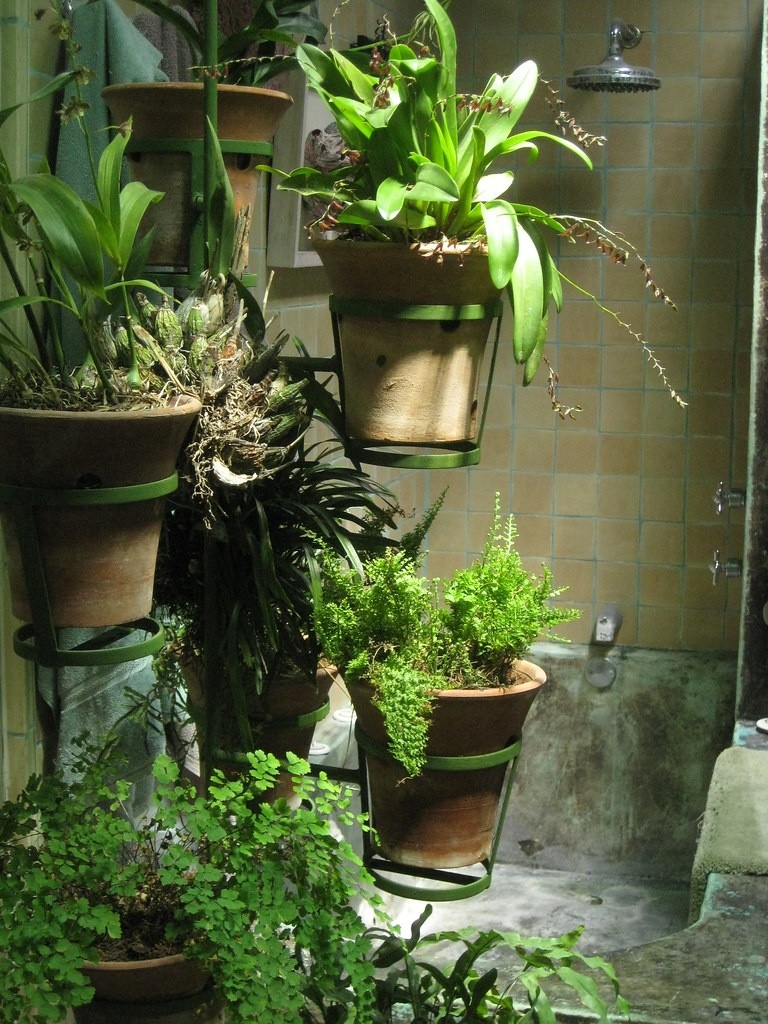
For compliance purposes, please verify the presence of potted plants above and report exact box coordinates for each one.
[0,0,688,1024]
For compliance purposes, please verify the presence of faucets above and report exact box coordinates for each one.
[596,603,623,642]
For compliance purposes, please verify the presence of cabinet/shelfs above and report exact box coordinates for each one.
[1,0,525,1024]
[266,61,347,271]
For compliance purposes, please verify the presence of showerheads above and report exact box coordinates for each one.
[564,52,661,96]
